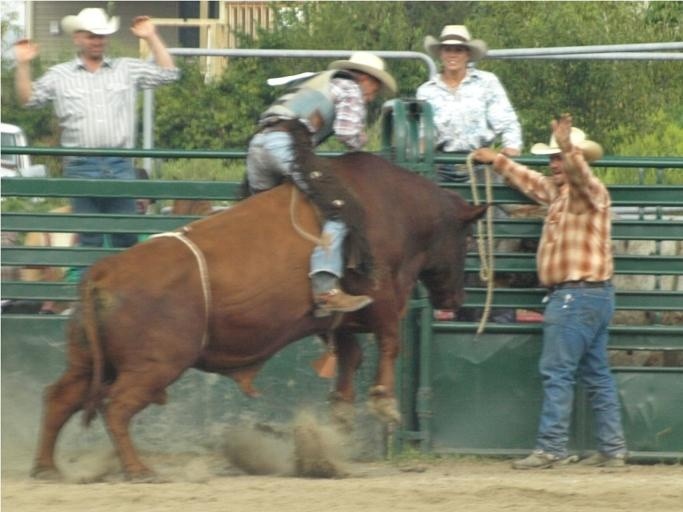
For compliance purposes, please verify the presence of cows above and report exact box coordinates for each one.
[29,147,495,484]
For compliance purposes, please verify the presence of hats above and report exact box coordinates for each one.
[421,24,489,64]
[527,120,609,163]
[328,48,400,99]
[57,8,123,38]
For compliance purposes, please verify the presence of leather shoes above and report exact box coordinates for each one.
[312,288,375,320]
[513,449,570,474]
[585,451,627,471]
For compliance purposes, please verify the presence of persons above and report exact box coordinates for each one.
[10,7,178,280]
[471,112,632,468]
[241,54,396,317]
[415,25,521,321]
